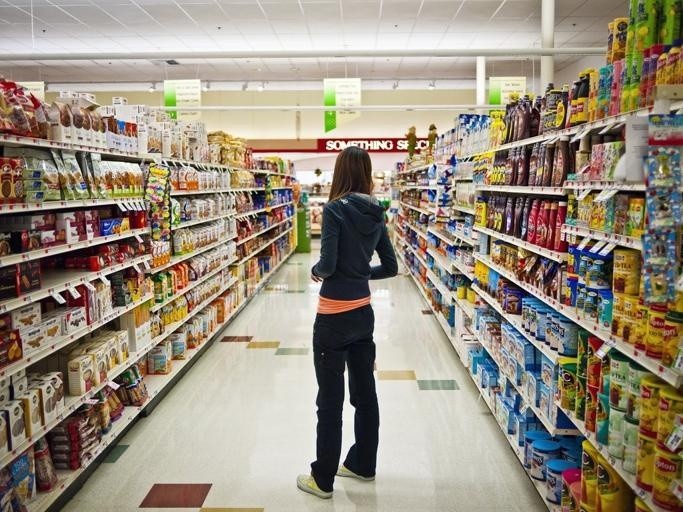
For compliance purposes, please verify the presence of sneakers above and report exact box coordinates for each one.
[336,465,376,481]
[297,473,333,497]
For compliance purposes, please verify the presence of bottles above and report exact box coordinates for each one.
[472,66,595,253]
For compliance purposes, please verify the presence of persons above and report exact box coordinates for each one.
[295,146,399,501]
[404,126,418,160]
[427,123,440,156]
